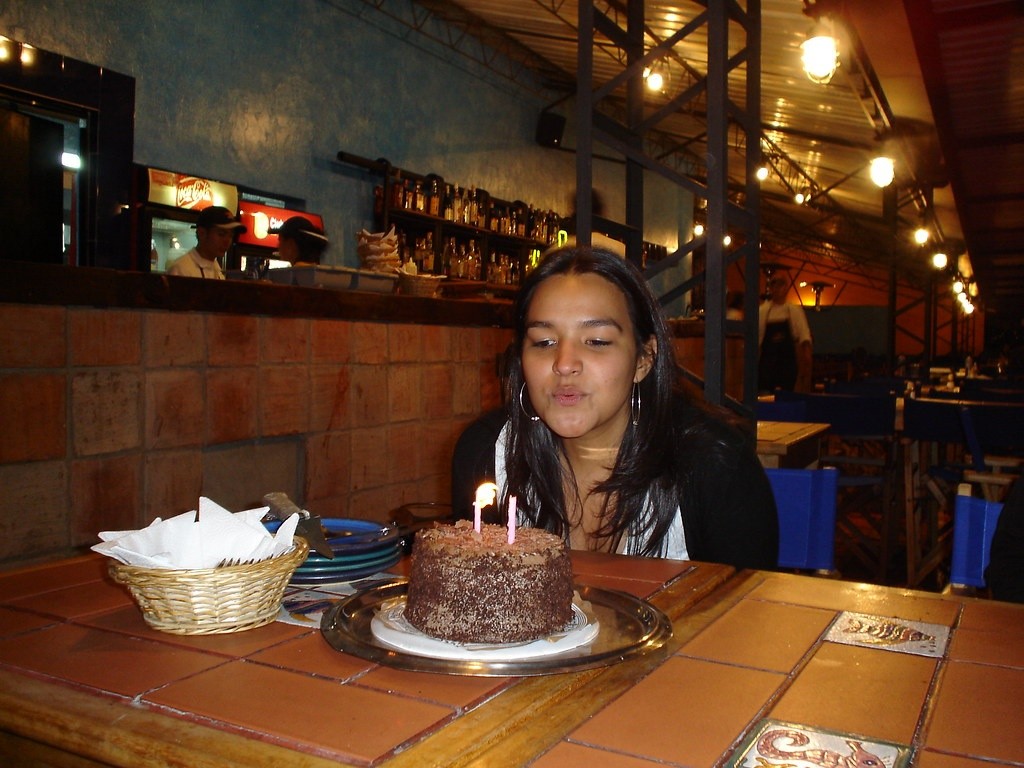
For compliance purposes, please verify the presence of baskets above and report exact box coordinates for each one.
[108,532,309,636]
[395,267,447,297]
[355,223,402,272]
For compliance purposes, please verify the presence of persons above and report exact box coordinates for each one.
[168,206,247,279]
[449,243,780,571]
[757,272,811,395]
[265,216,328,266]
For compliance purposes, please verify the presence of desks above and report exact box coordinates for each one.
[752,421,830,470]
[0,546,1024,768]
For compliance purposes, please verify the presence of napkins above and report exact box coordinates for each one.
[91,495,299,569]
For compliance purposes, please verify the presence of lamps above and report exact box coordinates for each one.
[798,17,840,84]
[866,149,897,188]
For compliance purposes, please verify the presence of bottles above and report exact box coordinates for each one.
[641,241,667,270]
[150,239,158,272]
[392,169,559,244]
[969,362,978,378]
[910,388,915,399]
[402,224,542,285]
[947,373,954,388]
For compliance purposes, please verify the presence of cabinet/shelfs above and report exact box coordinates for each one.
[334,148,677,300]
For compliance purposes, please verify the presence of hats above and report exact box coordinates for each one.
[268,216,328,241]
[190,206,247,234]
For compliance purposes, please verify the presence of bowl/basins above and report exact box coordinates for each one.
[445,281,482,293]
[487,282,520,297]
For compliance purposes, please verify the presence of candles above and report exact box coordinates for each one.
[507,495,516,545]
[473,482,498,533]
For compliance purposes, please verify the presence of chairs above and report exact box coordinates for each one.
[742,366,1023,597]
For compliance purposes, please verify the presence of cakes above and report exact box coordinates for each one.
[404,519,573,643]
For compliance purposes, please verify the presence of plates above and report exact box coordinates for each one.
[263,518,402,586]
